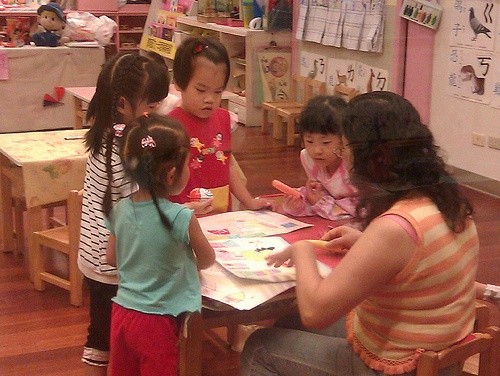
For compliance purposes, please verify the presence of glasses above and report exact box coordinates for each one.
[333,140,352,160]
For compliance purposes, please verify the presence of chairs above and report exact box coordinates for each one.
[11,190,83,306]
[417,302,500,376]
[262,73,355,148]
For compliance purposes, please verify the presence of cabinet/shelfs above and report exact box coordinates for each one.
[0,11,292,126]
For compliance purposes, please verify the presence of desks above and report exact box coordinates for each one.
[0,46,104,133]
[174,201,362,376]
[0,87,239,284]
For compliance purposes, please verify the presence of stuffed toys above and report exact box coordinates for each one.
[29,2,69,47]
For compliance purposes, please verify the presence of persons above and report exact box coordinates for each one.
[103,111,216,376]
[239,92,479,376]
[165,37,273,214]
[76,50,214,366]
[281,94,367,220]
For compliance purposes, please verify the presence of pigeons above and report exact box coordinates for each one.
[468,6,492,41]
